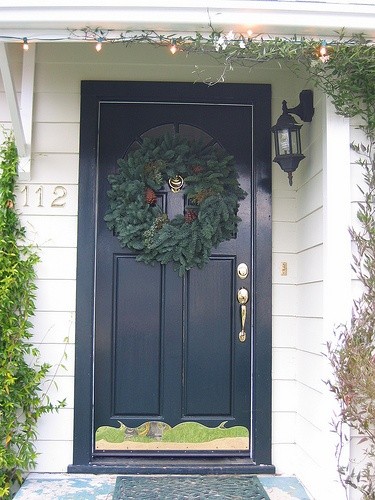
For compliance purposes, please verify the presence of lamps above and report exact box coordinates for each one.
[270,89,315,186]
